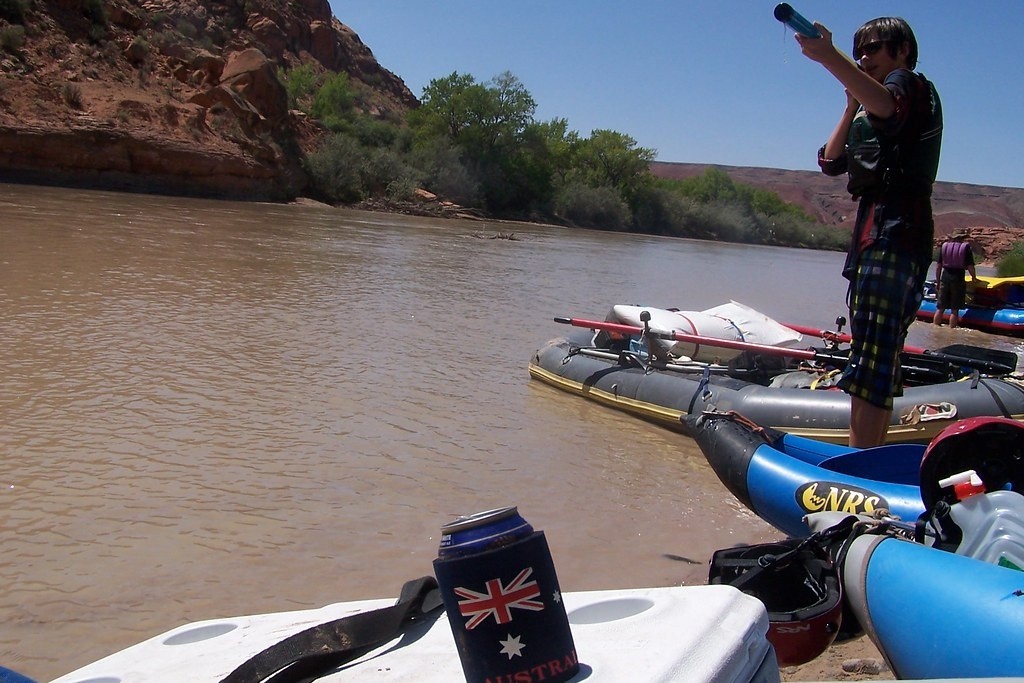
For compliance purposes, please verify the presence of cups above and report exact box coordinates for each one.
[433,529,579,683]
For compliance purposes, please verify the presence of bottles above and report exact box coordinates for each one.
[926,469,1024,571]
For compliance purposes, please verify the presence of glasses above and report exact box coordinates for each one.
[852,39,894,62]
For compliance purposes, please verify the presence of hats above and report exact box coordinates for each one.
[946,227,972,241]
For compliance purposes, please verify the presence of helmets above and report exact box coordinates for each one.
[706,543,842,669]
[915,411,1024,509]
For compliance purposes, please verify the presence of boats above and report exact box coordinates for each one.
[680,403,928,538]
[802,510,1024,679]
[917,284,1024,329]
[529,329,1024,446]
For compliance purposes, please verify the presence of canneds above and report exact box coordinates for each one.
[436,504,534,561]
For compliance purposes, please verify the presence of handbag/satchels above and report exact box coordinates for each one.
[843,109,899,197]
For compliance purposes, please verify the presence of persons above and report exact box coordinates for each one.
[772,0,945,451]
[932,227,978,329]
